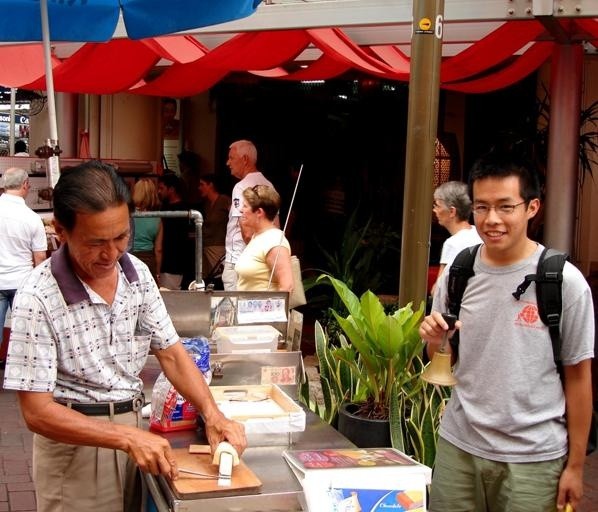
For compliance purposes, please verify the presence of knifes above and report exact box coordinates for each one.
[171,466,232,481]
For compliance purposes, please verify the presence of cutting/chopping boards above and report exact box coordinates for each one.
[160,444,263,502]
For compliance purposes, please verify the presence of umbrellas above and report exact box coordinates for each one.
[0,1,265,198]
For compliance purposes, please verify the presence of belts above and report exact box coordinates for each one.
[59,392,145,416]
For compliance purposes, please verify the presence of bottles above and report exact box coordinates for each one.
[18,124,29,137]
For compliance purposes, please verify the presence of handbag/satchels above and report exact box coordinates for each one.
[287,255,307,309]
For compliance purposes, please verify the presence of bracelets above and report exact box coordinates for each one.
[156,275,161,279]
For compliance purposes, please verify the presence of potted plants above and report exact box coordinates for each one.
[316,273,423,449]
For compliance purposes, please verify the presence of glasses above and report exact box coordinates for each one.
[252,184,259,201]
[473,200,526,215]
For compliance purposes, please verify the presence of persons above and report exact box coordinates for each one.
[2,161,247,512]
[430,181,483,297]
[0,166,48,368]
[14,140,31,158]
[281,368,292,384]
[234,185,293,327]
[128,178,164,287]
[419,149,595,511]
[158,174,189,290]
[221,140,280,292]
[198,174,231,279]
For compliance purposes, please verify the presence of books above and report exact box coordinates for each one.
[282,444,432,512]
